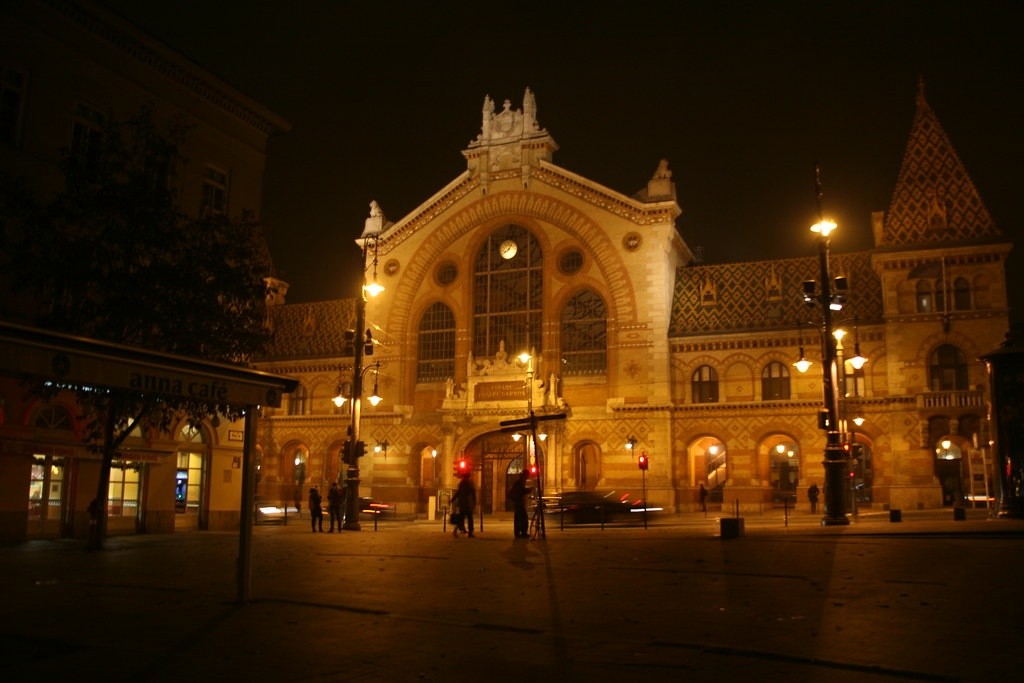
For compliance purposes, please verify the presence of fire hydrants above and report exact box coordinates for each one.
[85,498,101,554]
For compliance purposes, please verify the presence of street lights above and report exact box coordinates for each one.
[330,230,386,532]
[801,157,853,525]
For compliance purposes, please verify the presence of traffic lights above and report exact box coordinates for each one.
[459,460,467,479]
[637,454,648,470]
[529,466,538,476]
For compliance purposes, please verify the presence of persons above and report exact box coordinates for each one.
[450,473,476,538]
[807,482,819,514]
[512,469,533,539]
[699,484,706,512]
[293,490,302,509]
[308,484,326,533]
[327,482,345,533]
